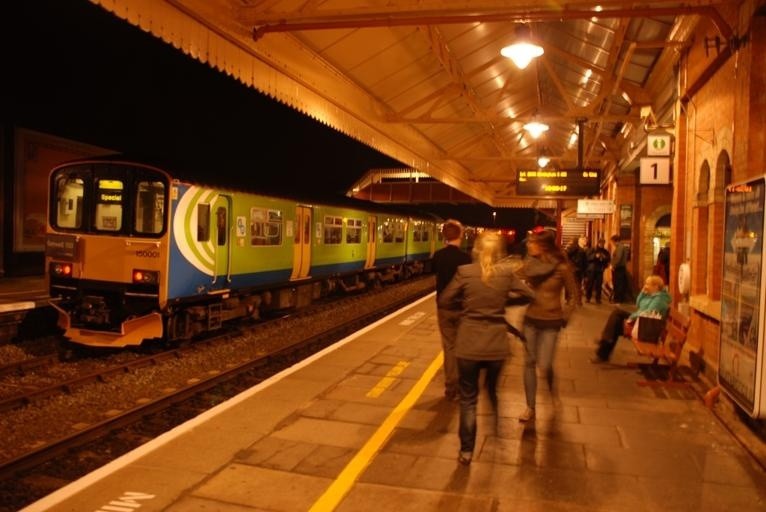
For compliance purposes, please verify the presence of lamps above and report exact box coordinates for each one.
[500,21,545,71]
[523,109,551,140]
[537,146,551,169]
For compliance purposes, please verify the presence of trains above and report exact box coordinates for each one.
[44,160,516,353]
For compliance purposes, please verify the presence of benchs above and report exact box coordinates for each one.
[631,307,689,386]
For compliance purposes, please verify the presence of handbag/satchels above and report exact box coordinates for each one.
[624,312,665,343]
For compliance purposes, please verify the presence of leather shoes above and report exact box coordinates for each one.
[458,449,473,465]
[520,406,535,421]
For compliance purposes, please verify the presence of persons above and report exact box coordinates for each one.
[433,229,541,469]
[563,232,671,305]
[514,224,579,428]
[424,215,473,404]
[586,273,674,367]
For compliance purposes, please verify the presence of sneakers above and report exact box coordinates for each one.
[566,297,602,306]
[591,339,609,364]
